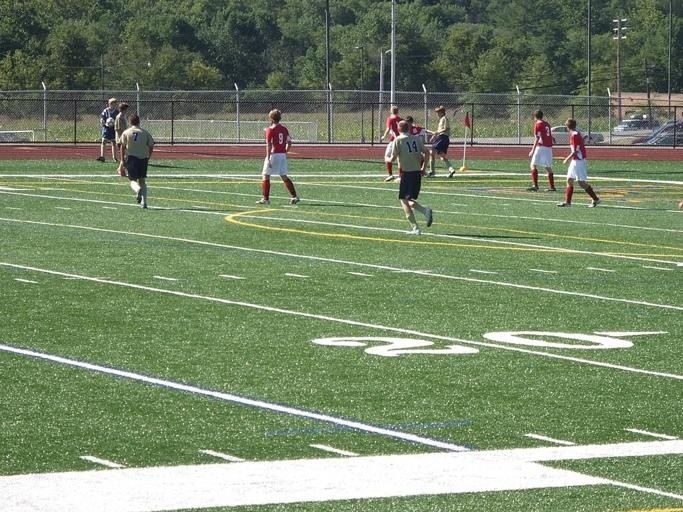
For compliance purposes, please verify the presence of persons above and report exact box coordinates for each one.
[380,104,456,181]
[256,109,300,204]
[385,120,433,236]
[526,109,555,192]
[556,118,601,208]
[96,97,130,162]
[116,115,154,207]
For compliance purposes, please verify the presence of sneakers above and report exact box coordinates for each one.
[290,197,300,204]
[544,188,556,192]
[588,197,601,208]
[384,175,394,182]
[255,198,270,204]
[447,168,455,178]
[556,203,571,207]
[406,229,421,235]
[425,209,432,227]
[526,186,539,191]
[96,156,147,209]
[426,170,435,175]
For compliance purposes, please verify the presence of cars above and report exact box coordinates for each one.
[538,125,604,146]
[633,131,683,146]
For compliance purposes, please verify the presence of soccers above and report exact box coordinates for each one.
[106,118,114,127]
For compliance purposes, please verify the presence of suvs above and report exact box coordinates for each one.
[631,119,682,146]
[613,114,659,133]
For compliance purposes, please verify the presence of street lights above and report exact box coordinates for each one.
[354,46,365,144]
